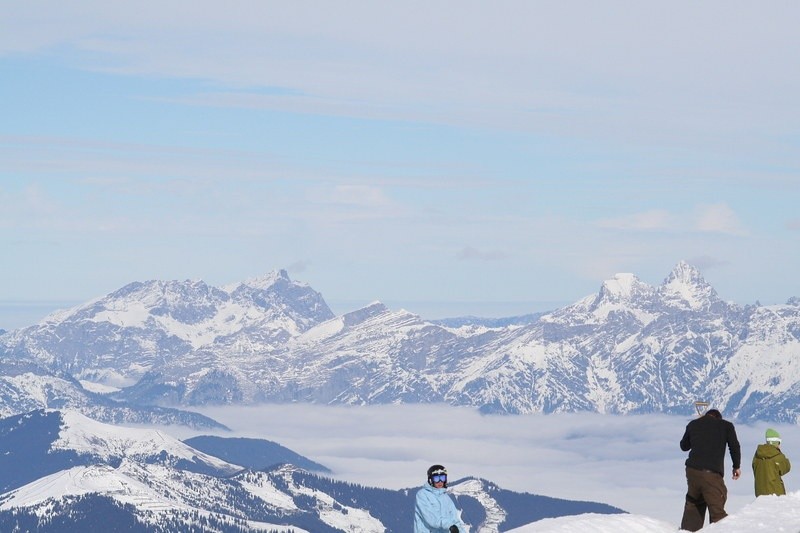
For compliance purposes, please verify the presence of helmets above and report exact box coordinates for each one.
[428,465,447,485]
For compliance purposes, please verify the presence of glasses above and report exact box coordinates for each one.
[432,474,446,482]
[778,439,782,445]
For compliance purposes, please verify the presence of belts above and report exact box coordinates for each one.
[696,467,712,472]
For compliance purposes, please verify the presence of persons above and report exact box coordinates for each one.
[414,465,467,533]
[680,409,742,532]
[752,428,792,498]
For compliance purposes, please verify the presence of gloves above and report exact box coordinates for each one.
[449,525,460,533]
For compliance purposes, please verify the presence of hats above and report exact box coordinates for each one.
[766,429,781,446]
